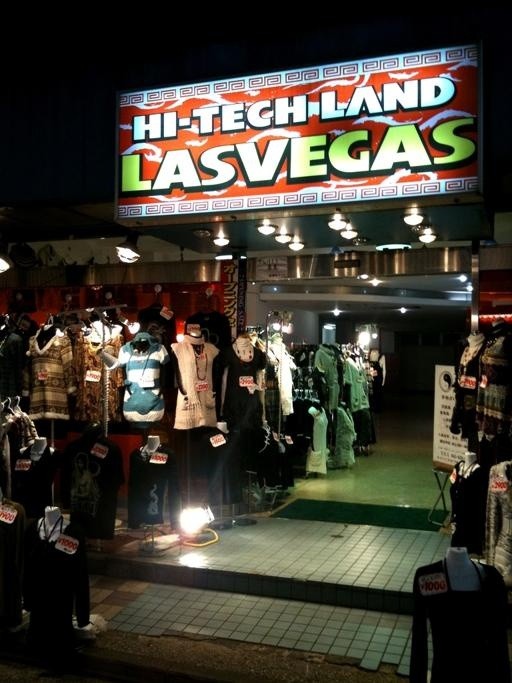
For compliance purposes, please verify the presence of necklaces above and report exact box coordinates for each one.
[196,352,208,380]
[235,343,252,359]
[42,517,62,542]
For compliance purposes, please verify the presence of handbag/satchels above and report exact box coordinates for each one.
[306,447,327,474]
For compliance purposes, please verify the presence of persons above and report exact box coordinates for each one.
[0,283,389,656]
[408,319,512,683]
